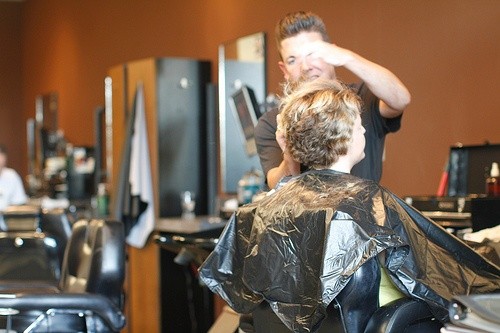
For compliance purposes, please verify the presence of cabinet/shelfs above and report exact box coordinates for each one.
[154,216,235,269]
[103,58,212,332]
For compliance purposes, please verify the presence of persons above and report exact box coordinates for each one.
[0,146,31,233]
[197,9,499,333]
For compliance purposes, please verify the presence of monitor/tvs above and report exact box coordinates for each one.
[228,87,263,156]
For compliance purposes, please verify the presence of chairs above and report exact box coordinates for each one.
[253,254,444,333]
[0,199,128,333]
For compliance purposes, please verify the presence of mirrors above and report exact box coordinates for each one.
[218,32,271,194]
[34,92,58,177]
